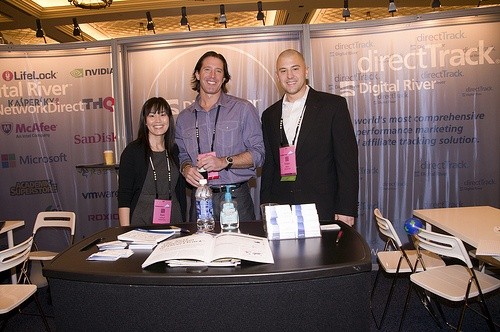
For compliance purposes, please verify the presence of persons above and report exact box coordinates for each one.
[174,51,264,223]
[118,97,187,227]
[261,50,358,227]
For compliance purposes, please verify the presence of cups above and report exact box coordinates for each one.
[260,203,279,232]
[103,151,114,165]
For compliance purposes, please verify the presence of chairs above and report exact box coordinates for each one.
[0,237,51,332]
[368,209,445,332]
[397,222,500,332]
[19,211,76,317]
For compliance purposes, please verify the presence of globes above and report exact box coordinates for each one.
[404,217,423,237]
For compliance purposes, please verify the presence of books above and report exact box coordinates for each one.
[141,233,275,269]
[118,230,175,249]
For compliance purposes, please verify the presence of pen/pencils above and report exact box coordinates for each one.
[336,231,343,242]
[170,225,190,232]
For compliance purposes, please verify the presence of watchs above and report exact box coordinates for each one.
[224,156,233,171]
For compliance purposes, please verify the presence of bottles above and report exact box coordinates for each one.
[195,179,215,232]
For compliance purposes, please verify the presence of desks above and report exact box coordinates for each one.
[411,205,500,281]
